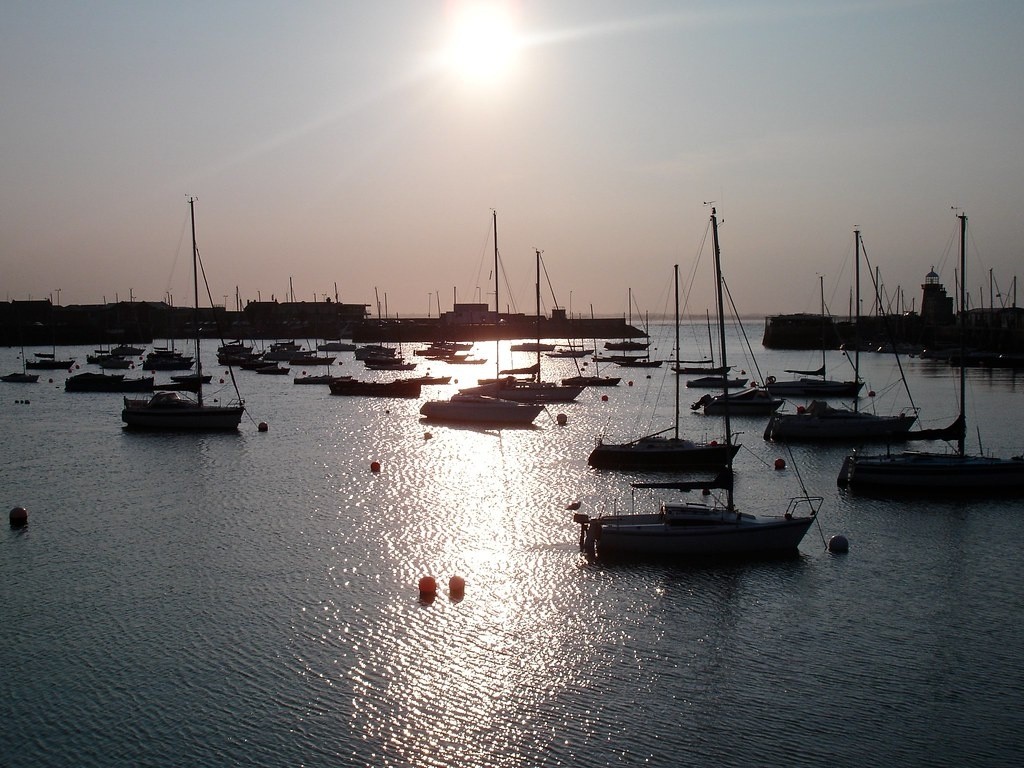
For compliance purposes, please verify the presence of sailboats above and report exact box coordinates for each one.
[0,344,40,383]
[566,203,825,570]
[23,195,1024,511]
